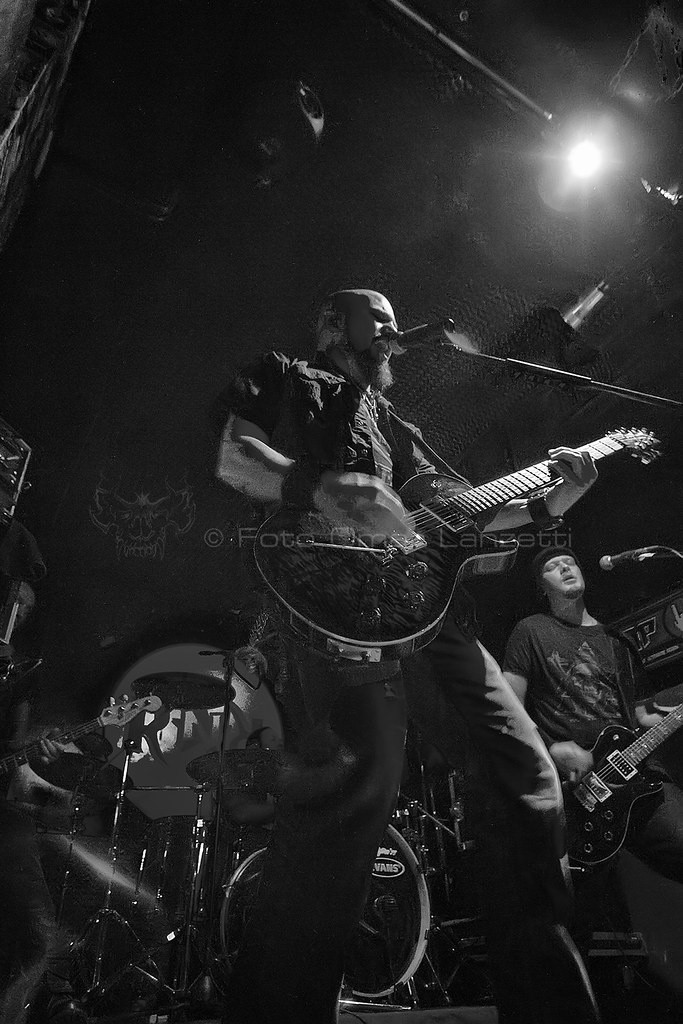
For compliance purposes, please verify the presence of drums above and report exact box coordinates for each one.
[140,813,433,1000]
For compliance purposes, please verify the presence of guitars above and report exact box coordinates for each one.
[0,691,163,780]
[561,698,683,866]
[246,425,663,665]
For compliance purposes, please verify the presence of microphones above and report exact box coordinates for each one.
[6,659,43,675]
[599,545,660,570]
[388,318,457,356]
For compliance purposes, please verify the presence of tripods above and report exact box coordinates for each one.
[60,736,240,1024]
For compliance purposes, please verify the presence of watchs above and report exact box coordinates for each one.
[528,486,564,532]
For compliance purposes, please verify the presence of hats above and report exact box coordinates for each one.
[532,547,582,590]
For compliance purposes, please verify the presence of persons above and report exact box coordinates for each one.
[501,544,683,936]
[0,517,54,976]
[201,287,601,1024]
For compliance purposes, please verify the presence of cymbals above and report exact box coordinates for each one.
[130,671,237,712]
[185,747,274,792]
